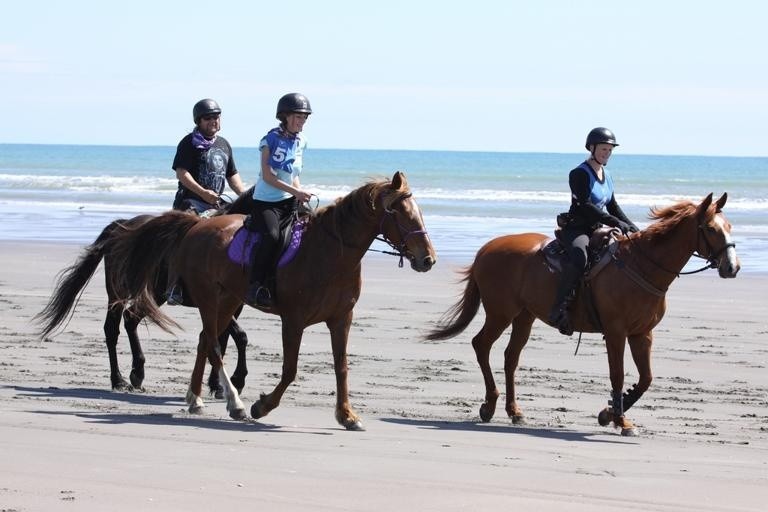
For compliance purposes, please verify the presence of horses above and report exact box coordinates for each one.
[31,183,259,396]
[85,169,439,432]
[417,189,744,438]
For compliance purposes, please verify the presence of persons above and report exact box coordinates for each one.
[244,92,313,310]
[164,98,244,306]
[548,126,640,336]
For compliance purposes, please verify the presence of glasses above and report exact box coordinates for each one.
[202,115,219,120]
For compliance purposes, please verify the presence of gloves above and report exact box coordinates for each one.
[617,220,640,233]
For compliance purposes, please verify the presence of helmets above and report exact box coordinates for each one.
[276,92,312,115]
[192,99,222,122]
[585,127,619,148]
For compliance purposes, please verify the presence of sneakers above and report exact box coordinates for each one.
[550,305,575,336]
[166,290,183,307]
[248,280,272,310]
